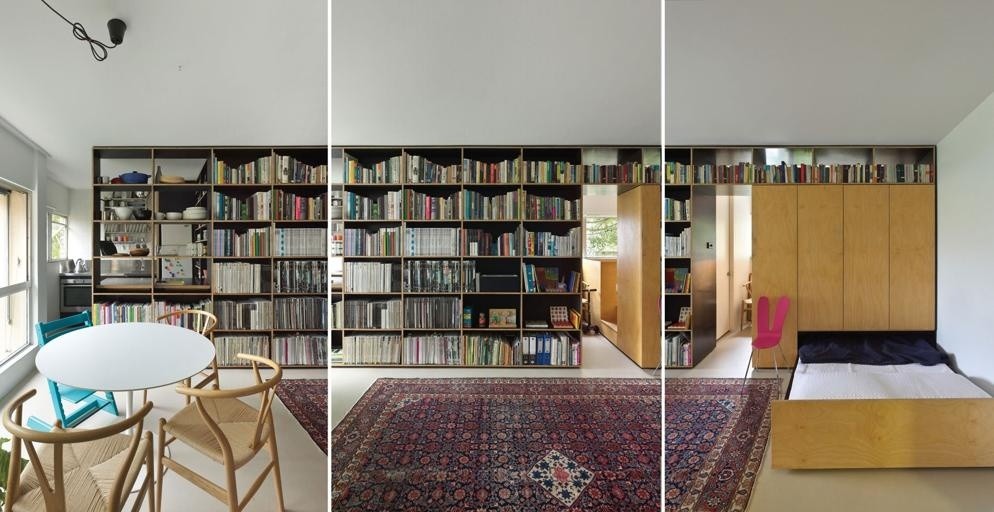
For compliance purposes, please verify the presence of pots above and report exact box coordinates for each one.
[117,171,152,184]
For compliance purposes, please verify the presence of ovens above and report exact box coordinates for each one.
[59,276,93,329]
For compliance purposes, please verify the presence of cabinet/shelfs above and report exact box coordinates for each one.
[330,144,661,370]
[90,144,327,369]
[665,144,937,370]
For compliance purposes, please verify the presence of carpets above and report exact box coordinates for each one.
[332,377,662,511]
[666,377,783,512]
[264,378,328,456]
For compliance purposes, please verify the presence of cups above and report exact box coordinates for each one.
[154,212,165,220]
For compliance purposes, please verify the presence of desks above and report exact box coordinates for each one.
[33,321,216,494]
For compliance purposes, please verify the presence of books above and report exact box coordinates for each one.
[92,147,936,369]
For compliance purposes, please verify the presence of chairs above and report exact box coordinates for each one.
[156,353,286,512]
[143,309,219,406]
[741,295,790,399]
[26,309,119,433]
[1,389,155,512]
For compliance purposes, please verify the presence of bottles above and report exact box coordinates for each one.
[154,165,161,184]
[68,259,76,274]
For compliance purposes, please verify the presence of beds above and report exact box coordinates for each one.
[770,330,994,469]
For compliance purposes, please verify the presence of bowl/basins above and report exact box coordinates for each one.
[113,207,151,220]
[183,207,207,220]
[165,211,182,220]
[160,176,186,184]
[129,247,149,255]
